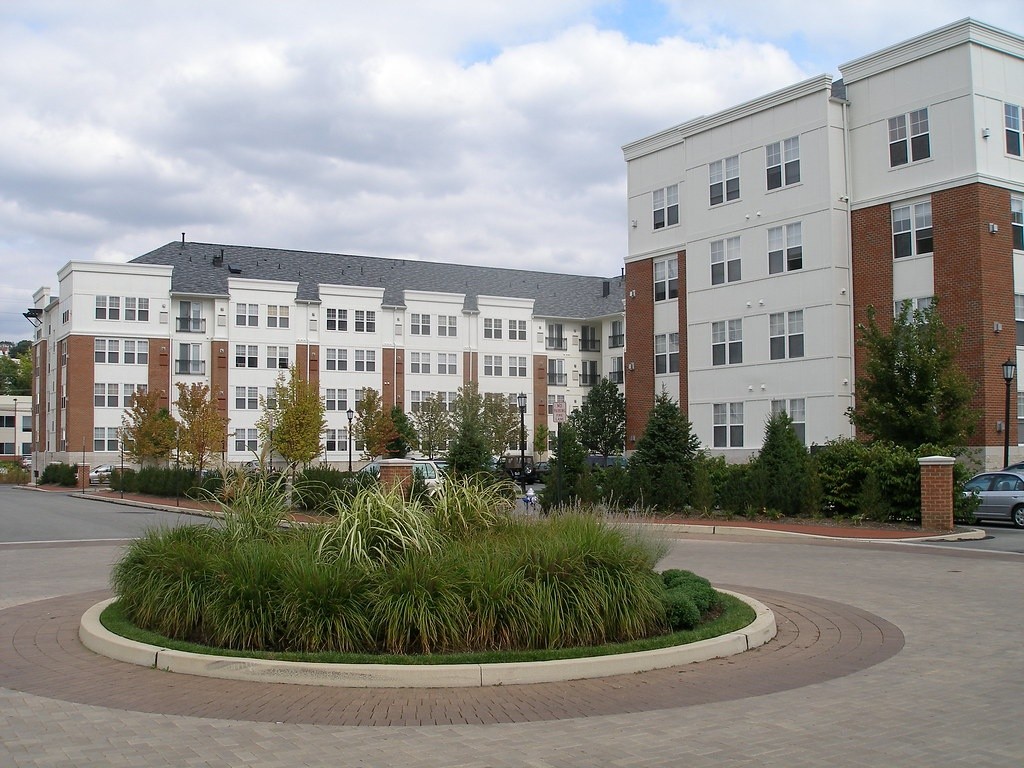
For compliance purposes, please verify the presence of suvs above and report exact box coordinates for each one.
[496,454,536,485]
[356,459,450,494]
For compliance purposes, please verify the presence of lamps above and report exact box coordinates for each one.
[629,434,636,442]
[631,219,637,228]
[629,362,635,371]
[630,289,637,299]
[994,321,1003,333]
[997,420,1005,433]
[989,222,999,234]
[982,127,990,139]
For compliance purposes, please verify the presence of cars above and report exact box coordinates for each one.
[954,471,1024,529]
[998,462,1024,476]
[74,464,131,486]
[534,461,550,481]
[244,460,275,473]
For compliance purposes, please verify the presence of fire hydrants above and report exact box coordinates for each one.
[523,488,537,511]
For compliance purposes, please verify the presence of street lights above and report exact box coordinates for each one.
[1001,355,1016,469]
[347,407,354,472]
[516,391,527,495]
